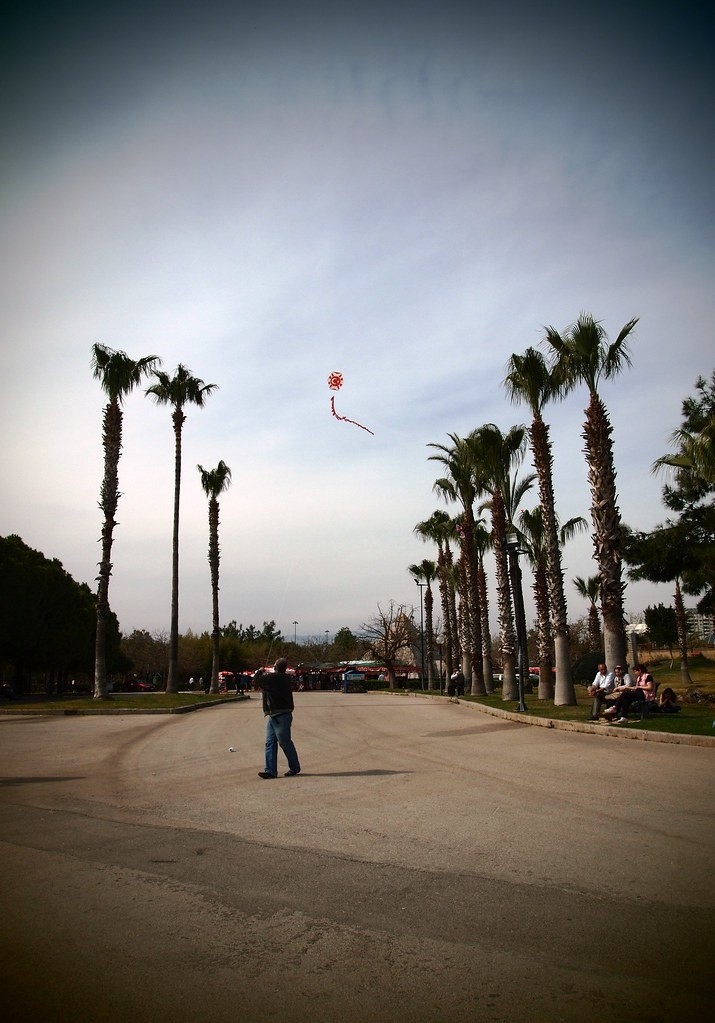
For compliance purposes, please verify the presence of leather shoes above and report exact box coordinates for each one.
[587,717,599,721]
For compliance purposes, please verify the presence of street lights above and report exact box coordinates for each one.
[292,620,299,644]
[504,531,529,711]
[415,578,427,693]
[436,635,444,695]
[325,630,330,644]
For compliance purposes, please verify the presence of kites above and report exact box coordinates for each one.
[328,371,375,436]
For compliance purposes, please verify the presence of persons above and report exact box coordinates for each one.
[198,676,206,691]
[377,672,385,681]
[189,676,194,691]
[447,668,465,697]
[604,665,633,723]
[253,657,301,779]
[660,688,682,713]
[297,673,305,692]
[587,663,615,720]
[604,663,656,723]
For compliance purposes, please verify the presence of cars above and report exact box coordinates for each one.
[451,672,539,683]
[120,679,157,692]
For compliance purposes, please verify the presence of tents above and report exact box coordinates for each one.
[218,660,418,690]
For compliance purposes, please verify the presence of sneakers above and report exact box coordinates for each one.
[604,707,617,713]
[258,771,277,778]
[618,717,627,723]
[283,770,297,776]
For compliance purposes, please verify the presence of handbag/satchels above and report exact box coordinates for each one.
[606,691,622,699]
